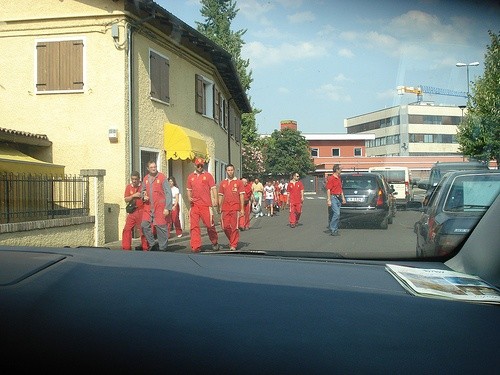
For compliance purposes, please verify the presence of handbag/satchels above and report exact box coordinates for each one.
[126,203,136,213]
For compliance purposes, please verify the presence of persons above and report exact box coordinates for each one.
[166,177,182,239]
[141,161,172,251]
[238,177,289,231]
[325,166,346,236]
[187,158,219,253]
[121,171,150,251]
[287,172,305,228]
[218,164,245,251]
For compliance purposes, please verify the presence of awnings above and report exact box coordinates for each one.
[163,122,210,164]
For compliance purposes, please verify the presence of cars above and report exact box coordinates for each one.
[327,160,499,259]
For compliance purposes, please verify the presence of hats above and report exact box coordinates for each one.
[195,157,204,165]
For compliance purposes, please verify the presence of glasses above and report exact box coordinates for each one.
[197,165,203,168]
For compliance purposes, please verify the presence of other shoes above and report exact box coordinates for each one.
[290,222,303,228]
[177,234,182,237]
[212,242,219,250]
[328,229,338,234]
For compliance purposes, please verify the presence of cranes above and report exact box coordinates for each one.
[398,85,475,103]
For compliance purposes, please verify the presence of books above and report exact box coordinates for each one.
[386,264,500,304]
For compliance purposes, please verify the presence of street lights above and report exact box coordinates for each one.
[455,61,480,107]
